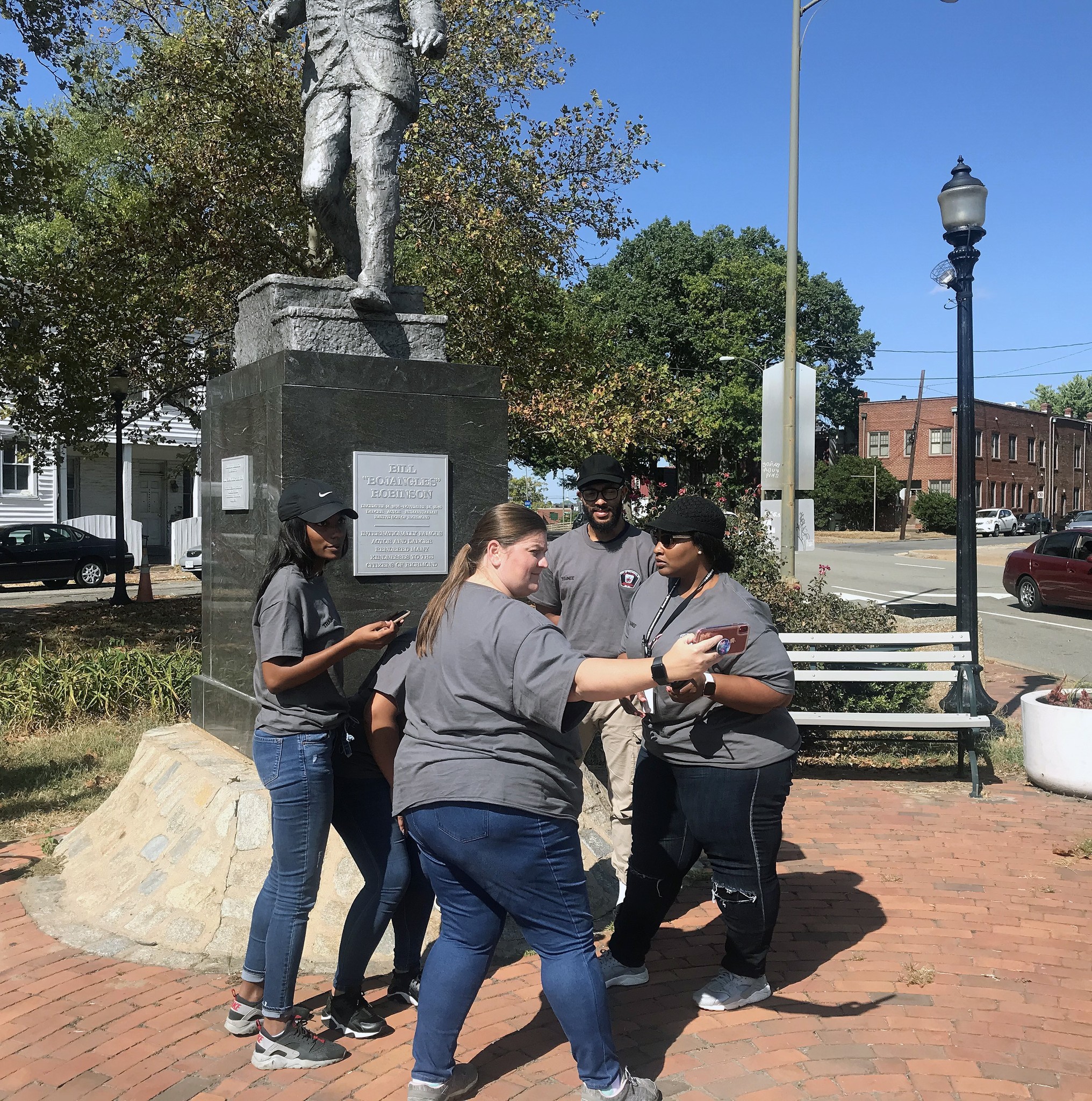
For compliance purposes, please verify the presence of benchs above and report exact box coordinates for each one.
[778,631,990,798]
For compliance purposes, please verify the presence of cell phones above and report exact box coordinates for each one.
[693,622,751,656]
[670,679,689,690]
[374,610,410,631]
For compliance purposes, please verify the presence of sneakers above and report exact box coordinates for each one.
[320,991,387,1039]
[387,968,424,1007]
[580,1066,661,1101]
[408,1063,479,1101]
[596,949,649,989]
[252,1015,347,1071]
[692,967,771,1011]
[224,989,310,1035]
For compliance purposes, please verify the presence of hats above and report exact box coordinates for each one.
[278,479,359,523]
[576,454,624,486]
[643,496,726,539]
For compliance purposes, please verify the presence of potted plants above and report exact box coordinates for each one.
[1020,669,1092,801]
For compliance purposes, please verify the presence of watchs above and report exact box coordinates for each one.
[702,672,716,700]
[650,656,673,686]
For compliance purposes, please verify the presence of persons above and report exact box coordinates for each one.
[261,0,453,313]
[387,502,728,1101]
[220,480,398,1070]
[326,626,433,1040]
[520,451,668,918]
[591,497,803,1012]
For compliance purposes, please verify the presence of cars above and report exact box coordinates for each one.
[1014,513,1052,536]
[180,545,202,581]
[1065,510,1092,531]
[1002,529,1092,613]
[0,524,135,588]
[1056,510,1089,532]
[976,509,1018,537]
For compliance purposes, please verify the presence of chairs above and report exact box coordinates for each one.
[1078,540,1092,560]
[20,534,31,545]
[6,537,17,546]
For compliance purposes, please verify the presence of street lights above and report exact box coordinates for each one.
[929,154,996,719]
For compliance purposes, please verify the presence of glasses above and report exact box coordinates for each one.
[580,483,624,501]
[650,530,692,548]
